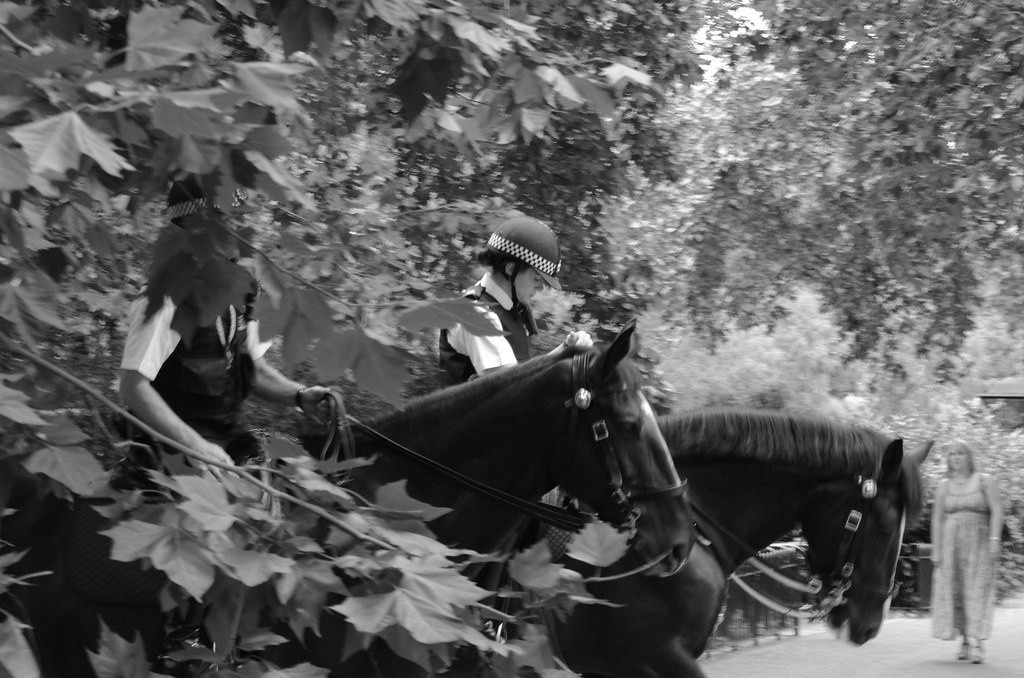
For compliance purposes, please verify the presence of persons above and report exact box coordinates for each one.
[63,177,332,678]
[929,439,1004,667]
[439,216,595,646]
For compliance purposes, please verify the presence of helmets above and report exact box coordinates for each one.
[476,217,564,289]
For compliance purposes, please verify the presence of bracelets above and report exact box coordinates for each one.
[294,387,304,408]
[989,537,1003,542]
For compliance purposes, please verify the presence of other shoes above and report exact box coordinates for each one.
[957,643,982,664]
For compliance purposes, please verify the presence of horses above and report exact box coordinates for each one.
[0,318,694,678]
[329,412,934,678]
